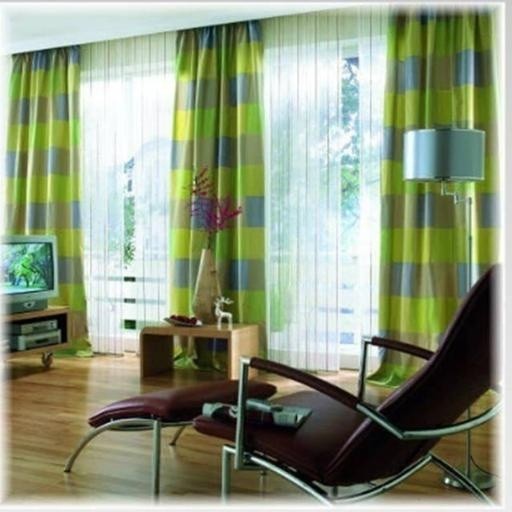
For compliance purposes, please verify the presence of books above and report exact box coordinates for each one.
[202,400,313,428]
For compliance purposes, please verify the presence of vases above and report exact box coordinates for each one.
[190,247,223,325]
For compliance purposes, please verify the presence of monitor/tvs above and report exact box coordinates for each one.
[0,235,59,314]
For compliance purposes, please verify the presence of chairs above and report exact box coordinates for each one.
[191,261,498,504]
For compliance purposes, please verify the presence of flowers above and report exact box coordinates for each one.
[181,164,244,251]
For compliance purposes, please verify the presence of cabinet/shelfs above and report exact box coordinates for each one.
[1,304,74,372]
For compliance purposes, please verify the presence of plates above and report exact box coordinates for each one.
[163,315,204,327]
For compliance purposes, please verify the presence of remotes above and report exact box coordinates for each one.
[246,398,284,412]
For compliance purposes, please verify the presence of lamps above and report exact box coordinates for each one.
[399,124,496,494]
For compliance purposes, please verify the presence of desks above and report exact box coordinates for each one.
[137,322,261,382]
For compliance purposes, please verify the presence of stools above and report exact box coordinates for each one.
[63,380,279,499]
